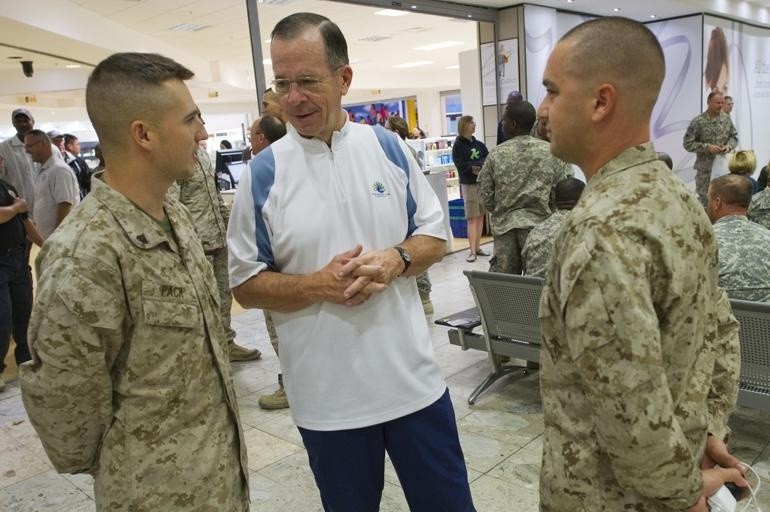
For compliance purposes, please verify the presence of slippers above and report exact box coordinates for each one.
[476,249,490,256]
[467,253,477,262]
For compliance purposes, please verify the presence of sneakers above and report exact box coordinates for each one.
[228,339,261,362]
[259,374,289,410]
[0,377,5,393]
[420,291,434,315]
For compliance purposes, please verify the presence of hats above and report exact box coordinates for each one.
[46,130,65,139]
[12,109,33,119]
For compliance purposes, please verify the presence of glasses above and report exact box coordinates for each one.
[271,66,344,94]
[23,140,43,147]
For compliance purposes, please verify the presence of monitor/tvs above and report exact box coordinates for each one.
[216,149,244,171]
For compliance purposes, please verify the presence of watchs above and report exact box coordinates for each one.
[392,243,412,276]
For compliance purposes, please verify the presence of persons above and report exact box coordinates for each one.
[411,125,426,140]
[703,24,731,96]
[214,8,479,512]
[1,108,105,394]
[656,91,770,303]
[382,112,437,314]
[166,87,291,409]
[452,90,586,279]
[14,48,259,512]
[531,11,753,511]
